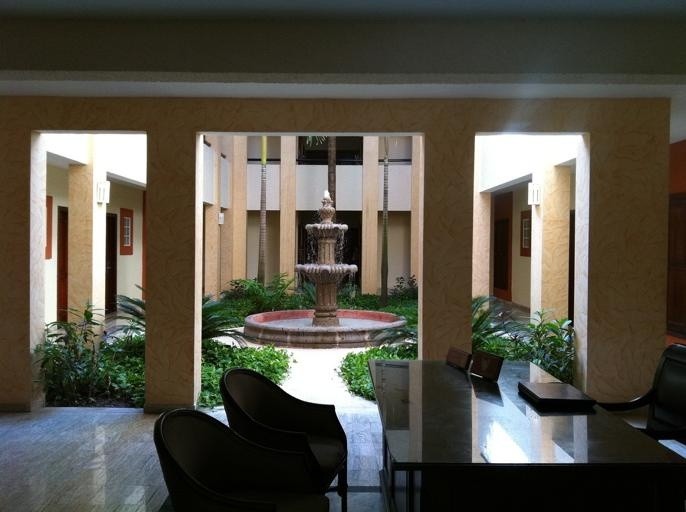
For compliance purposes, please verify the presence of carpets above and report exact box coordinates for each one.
[157,486,385,512]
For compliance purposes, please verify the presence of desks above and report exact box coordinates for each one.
[366,359,685,512]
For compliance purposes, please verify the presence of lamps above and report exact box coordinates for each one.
[526,182,540,208]
[218,212,224,225]
[96,180,110,204]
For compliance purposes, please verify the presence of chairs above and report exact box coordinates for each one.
[152,367,350,512]
[596,342,686,447]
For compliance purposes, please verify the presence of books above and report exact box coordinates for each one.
[517,379,598,411]
